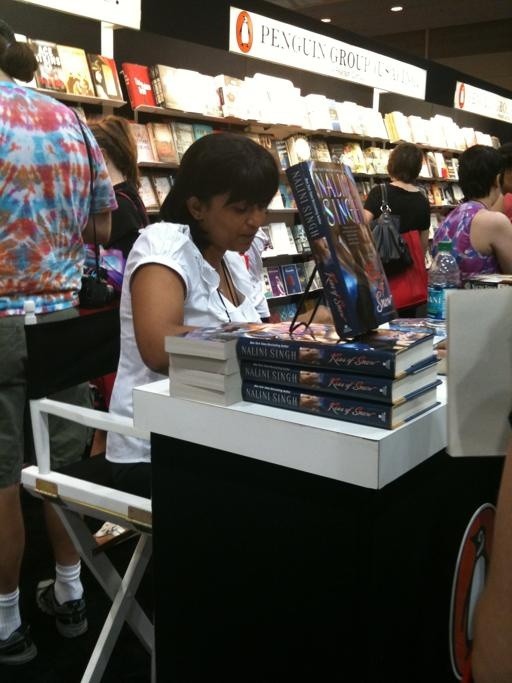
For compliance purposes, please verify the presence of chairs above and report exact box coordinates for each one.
[20,300,156,683]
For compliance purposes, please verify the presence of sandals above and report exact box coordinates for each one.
[93,521,133,547]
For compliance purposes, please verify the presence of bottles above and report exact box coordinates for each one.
[427,238,460,346]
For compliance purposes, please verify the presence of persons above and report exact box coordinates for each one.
[101,131,281,502]
[489,144,512,228]
[428,141,511,278]
[0,16,121,671]
[458,412,511,681]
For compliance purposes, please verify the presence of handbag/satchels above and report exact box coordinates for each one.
[369,212,412,276]
[78,241,126,310]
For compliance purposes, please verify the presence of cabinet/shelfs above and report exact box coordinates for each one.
[0,0,512,323]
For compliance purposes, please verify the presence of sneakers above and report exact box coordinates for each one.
[0,620,38,666]
[28,584,89,638]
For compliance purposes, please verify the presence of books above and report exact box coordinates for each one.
[17,31,501,324]
[233,379,445,430]
[233,317,440,380]
[237,355,439,406]
